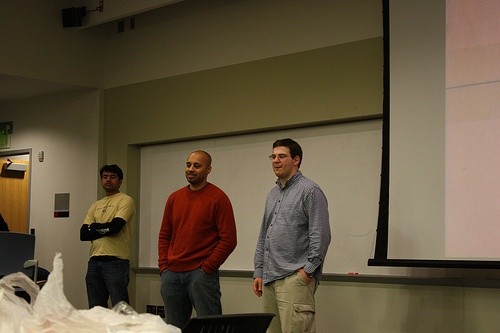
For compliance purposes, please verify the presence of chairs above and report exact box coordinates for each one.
[0,226,52,293]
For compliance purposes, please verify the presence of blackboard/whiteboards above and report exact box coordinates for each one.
[133,114,500,289]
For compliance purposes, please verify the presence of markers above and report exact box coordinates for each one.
[348,272,358,275]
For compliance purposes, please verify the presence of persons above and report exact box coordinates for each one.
[156,148,237,325]
[78,162,136,310]
[0,214,8,232]
[252,137,332,332]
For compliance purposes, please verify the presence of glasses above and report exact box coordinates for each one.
[269,155,294,160]
